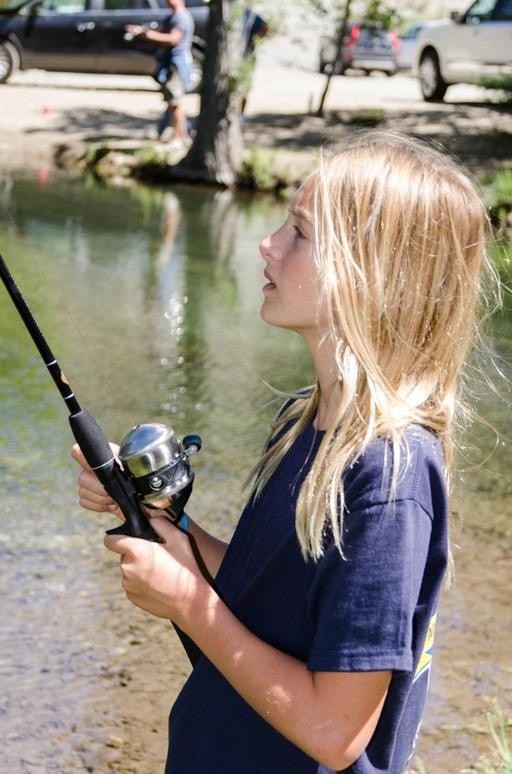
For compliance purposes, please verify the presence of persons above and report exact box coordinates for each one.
[125,0,196,154]
[141,196,182,360]
[236,1,269,116]
[71,127,512,774]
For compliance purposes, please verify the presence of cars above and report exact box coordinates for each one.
[318,0,512,101]
[0,0,246,84]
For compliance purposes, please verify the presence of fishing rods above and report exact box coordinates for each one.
[76,18,132,29]
[0,255,201,669]
